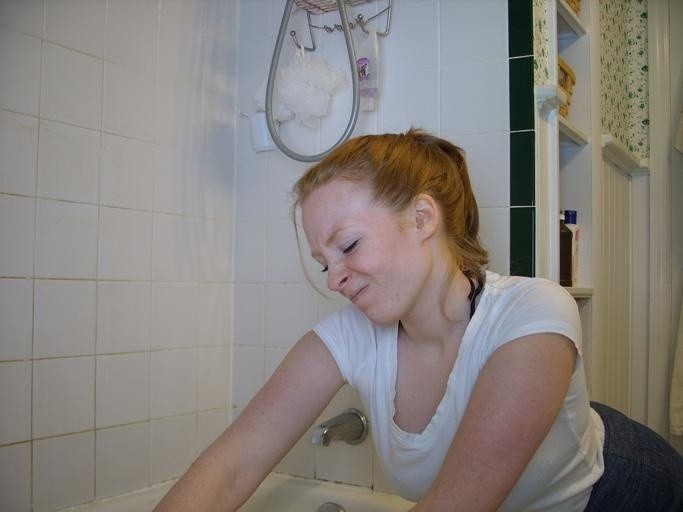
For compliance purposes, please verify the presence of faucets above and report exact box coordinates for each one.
[311,407,368,447]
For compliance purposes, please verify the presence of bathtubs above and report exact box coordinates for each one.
[60,472,417,512]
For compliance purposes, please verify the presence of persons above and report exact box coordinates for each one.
[150,130,683,512]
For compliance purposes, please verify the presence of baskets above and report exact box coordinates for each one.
[558,55,576,118]
